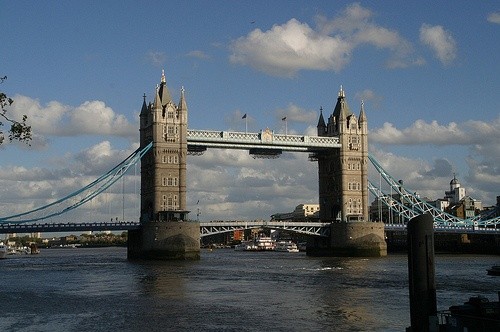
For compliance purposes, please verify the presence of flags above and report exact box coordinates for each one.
[242,114,246,119]
[281,116,286,120]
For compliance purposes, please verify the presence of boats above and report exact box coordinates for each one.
[272,241,299,253]
[406,295,500,332]
[234,236,276,252]
